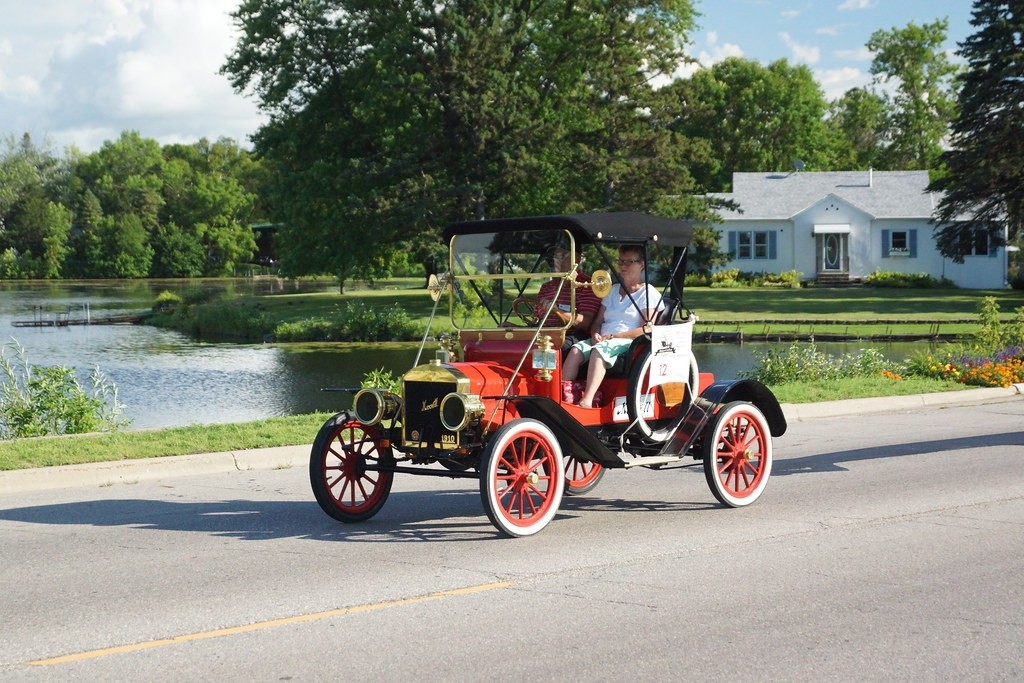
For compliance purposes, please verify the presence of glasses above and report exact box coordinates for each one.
[615,259,640,267]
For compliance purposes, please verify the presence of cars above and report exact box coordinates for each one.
[307,209,790,540]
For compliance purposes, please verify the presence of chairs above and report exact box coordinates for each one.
[581,296,681,377]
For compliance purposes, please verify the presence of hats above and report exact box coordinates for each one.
[547,236,582,256]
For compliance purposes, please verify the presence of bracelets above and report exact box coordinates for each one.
[609,334,613,340]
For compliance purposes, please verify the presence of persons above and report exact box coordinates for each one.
[562,245,666,408]
[498,238,602,367]
